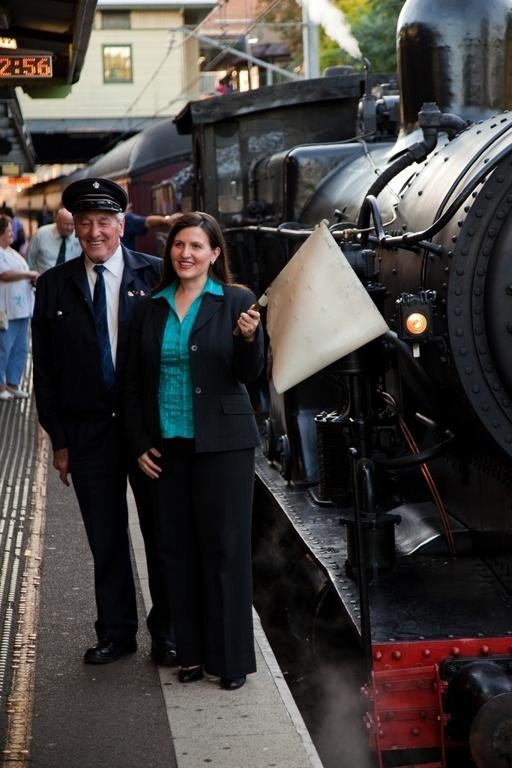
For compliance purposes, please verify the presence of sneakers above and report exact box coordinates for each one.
[0,386,29,400]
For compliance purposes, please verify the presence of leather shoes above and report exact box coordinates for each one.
[84,638,246,691]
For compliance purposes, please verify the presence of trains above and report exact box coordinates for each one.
[17,0,512,768]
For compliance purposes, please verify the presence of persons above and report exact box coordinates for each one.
[0,194,184,402]
[125,211,270,690]
[28,176,179,667]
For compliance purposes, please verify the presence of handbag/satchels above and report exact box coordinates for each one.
[0,311,9,330]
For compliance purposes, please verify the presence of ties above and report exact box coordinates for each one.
[56,236,68,266]
[91,264,116,393]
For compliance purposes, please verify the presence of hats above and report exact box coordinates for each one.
[62,177,128,214]
